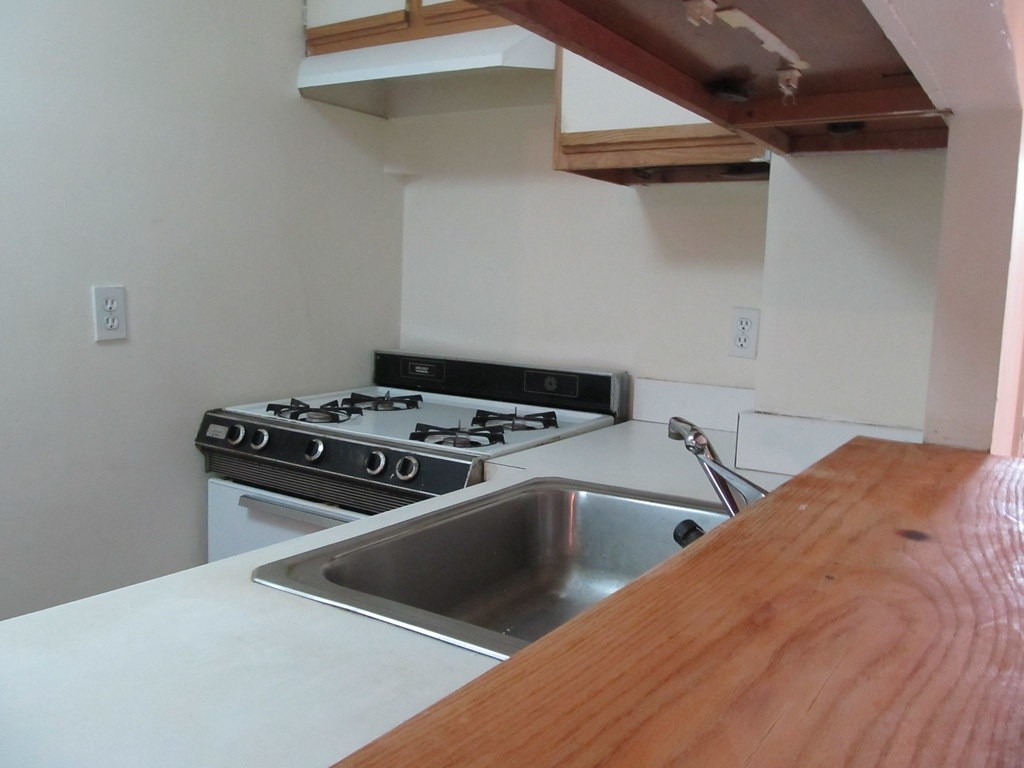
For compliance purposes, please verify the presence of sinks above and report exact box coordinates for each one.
[248,475,750,663]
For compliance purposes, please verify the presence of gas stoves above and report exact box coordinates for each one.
[192,346,631,517]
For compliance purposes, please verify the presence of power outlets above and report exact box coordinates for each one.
[91,285,129,345]
[726,306,760,363]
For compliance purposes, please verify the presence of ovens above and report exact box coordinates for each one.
[206,476,370,566]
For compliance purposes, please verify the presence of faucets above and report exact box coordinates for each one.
[670,412,770,548]
